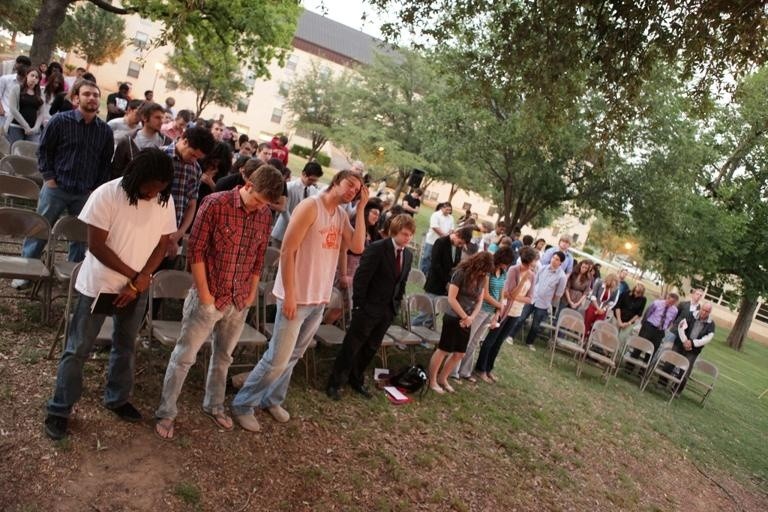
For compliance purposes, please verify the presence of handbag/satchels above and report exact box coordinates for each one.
[389,363,430,396]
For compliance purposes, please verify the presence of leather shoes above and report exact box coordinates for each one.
[327,386,341,400]
[356,385,372,398]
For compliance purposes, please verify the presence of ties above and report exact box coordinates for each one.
[395,249,402,279]
[661,306,668,328]
[602,286,608,301]
[304,186,307,197]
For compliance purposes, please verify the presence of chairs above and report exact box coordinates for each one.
[379,336,394,377]
[229,283,267,376]
[403,293,442,365]
[146,271,212,395]
[313,286,347,382]
[409,269,426,287]
[386,325,423,366]
[0,123,140,370]
[434,296,457,334]
[264,278,308,387]
[260,249,283,273]
[530,291,719,407]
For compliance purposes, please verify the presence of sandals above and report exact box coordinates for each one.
[431,373,499,394]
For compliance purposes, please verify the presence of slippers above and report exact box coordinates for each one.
[154,419,176,441]
[201,409,235,431]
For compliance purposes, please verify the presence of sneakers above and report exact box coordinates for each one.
[45,414,67,440]
[111,402,142,421]
[11,278,33,290]
[528,344,536,351]
[268,406,290,423]
[506,336,513,345]
[236,414,260,432]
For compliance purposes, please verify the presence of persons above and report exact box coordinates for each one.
[1,56,715,441]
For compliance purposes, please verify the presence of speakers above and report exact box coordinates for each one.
[407,168,426,189]
